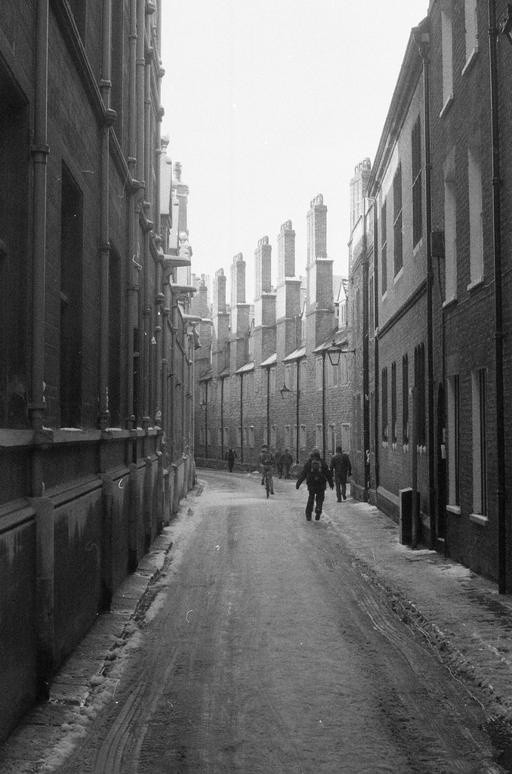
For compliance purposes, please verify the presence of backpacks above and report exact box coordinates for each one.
[262,451,273,465]
[309,458,323,481]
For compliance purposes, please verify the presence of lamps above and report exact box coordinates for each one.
[280,382,297,400]
[326,341,355,366]
[199,399,208,412]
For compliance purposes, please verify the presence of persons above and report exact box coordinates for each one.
[225,446,237,473]
[259,445,293,495]
[330,446,352,503]
[296,448,334,521]
